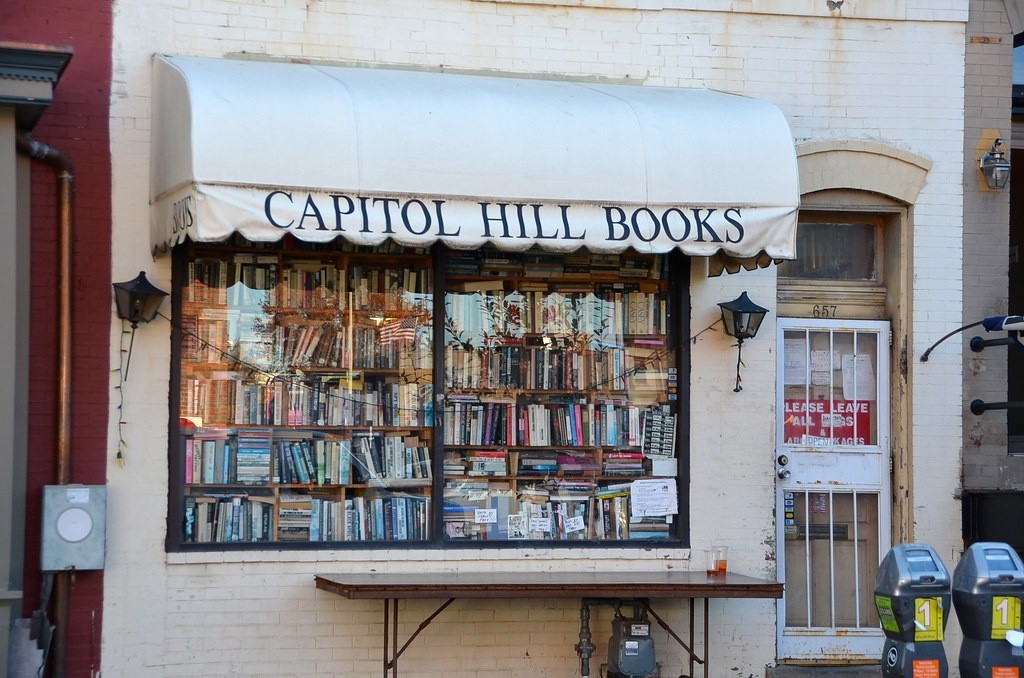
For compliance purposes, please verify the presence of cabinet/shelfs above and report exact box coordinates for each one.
[182,243,674,542]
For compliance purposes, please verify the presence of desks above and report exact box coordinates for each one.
[313,571,787,678]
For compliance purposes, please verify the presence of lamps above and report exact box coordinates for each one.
[979,138,1011,190]
[112,270,170,382]
[717,291,769,392]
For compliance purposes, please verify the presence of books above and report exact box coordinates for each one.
[182,253,678,542]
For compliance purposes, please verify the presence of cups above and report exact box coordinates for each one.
[711,545,729,577]
[703,549,722,577]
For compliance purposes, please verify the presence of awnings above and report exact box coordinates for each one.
[151,56,799,277]
